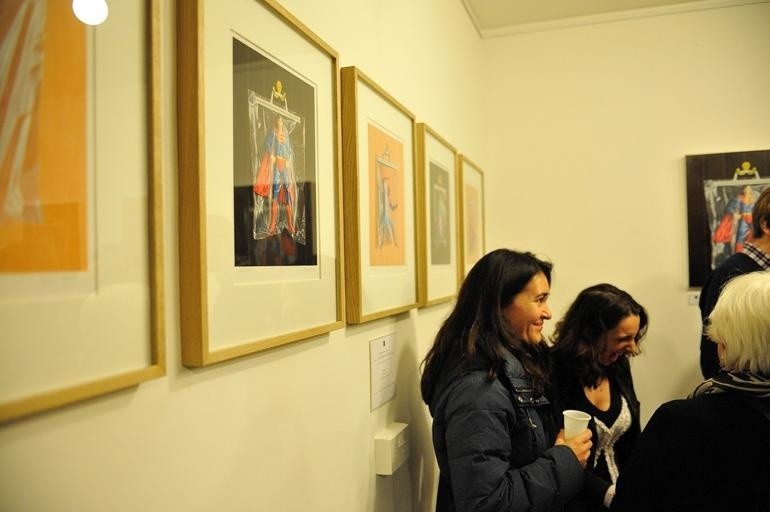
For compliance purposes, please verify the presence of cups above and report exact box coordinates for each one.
[562,409,591,440]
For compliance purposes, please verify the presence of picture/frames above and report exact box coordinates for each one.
[177,1,345,370]
[2,1,168,422]
[338,64,425,325]
[416,121,459,309]
[457,153,485,297]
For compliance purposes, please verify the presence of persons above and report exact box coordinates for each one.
[697,187,770,378]
[548,281,651,511]
[604,268,770,511]
[377,177,399,247]
[420,247,596,511]
[725,185,756,254]
[265,115,296,237]
